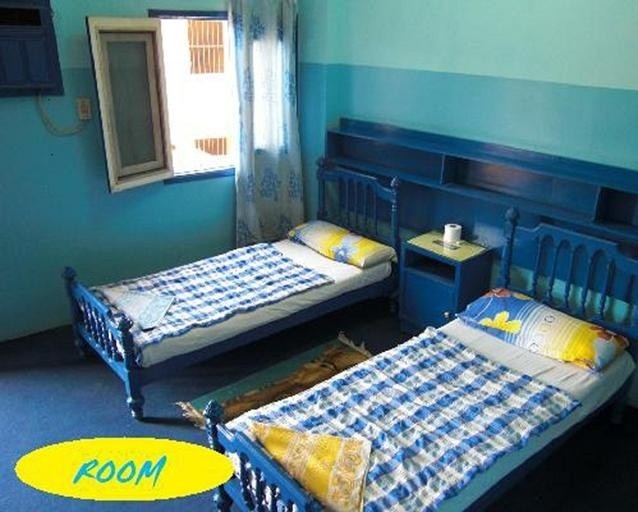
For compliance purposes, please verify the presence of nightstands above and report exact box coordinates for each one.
[400,227,493,332]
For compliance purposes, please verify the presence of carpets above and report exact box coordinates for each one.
[171,329,374,434]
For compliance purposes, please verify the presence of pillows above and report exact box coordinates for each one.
[455,286,631,371]
[287,217,396,268]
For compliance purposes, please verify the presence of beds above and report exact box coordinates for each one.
[61,156,401,422]
[205,206,638,510]
